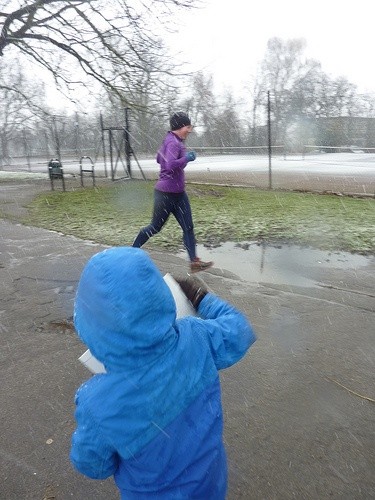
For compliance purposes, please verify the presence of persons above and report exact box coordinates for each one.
[71,247,257,500]
[134,112,216,274]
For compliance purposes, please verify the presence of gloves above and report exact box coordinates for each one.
[175,276,207,308]
[186,152,195,161]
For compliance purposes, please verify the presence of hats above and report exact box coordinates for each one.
[170,112,191,130]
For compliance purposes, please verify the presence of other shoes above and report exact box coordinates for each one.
[190,258,213,274]
